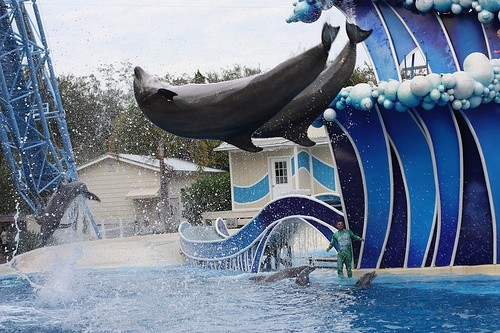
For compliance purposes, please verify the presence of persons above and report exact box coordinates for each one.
[326,220,366,279]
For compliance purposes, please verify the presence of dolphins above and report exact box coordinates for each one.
[296,266,316,286]
[352,271,378,288]
[251,20,373,148]
[249,266,307,285]
[132,22,341,152]
[31,179,101,248]
